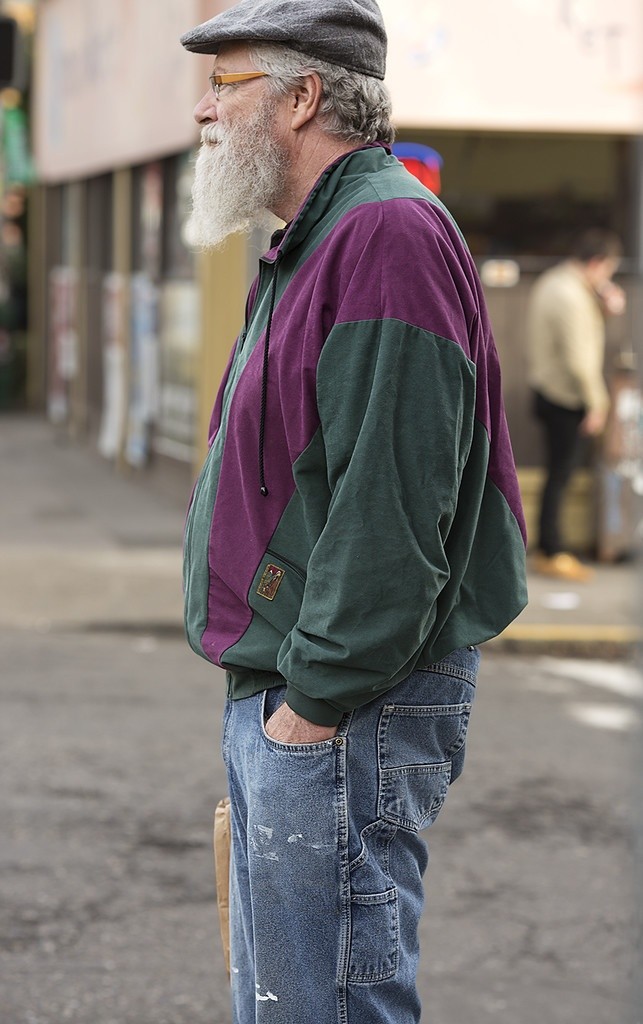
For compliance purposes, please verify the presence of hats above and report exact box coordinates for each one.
[179,0,386,80]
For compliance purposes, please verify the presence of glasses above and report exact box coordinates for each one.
[209,73,266,101]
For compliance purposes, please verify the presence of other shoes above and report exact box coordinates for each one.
[536,552,593,582]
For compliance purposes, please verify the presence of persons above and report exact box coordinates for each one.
[526,225,625,578]
[172,2,532,1024]
